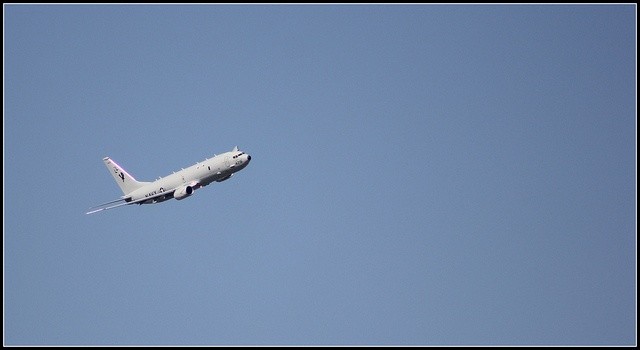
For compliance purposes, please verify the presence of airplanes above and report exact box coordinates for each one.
[86,145,251,219]
